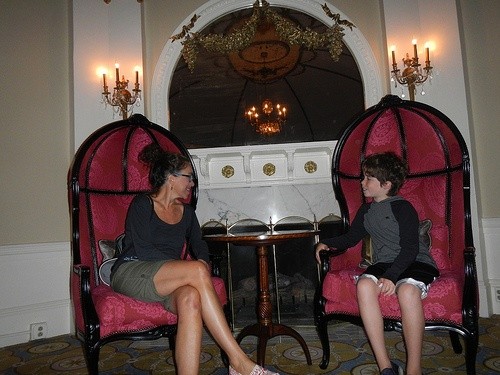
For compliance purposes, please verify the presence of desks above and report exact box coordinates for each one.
[203,228,321,372]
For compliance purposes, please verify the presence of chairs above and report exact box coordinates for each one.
[67,113,231,375]
[312,94,479,375]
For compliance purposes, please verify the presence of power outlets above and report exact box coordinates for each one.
[30,321,48,340]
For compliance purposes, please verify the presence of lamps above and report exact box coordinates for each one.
[241,51,290,137]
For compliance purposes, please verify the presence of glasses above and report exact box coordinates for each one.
[172,173,193,183]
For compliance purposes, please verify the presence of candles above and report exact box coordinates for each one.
[390,42,398,67]
[424,41,431,66]
[134,66,140,89]
[412,38,419,62]
[114,63,120,87]
[101,67,108,92]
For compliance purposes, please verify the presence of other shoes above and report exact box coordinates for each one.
[229,365,279,375]
[380,362,403,375]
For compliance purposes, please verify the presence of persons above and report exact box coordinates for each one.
[315,150,440,375]
[110,141,279,375]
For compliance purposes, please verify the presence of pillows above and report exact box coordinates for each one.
[361,218,435,271]
